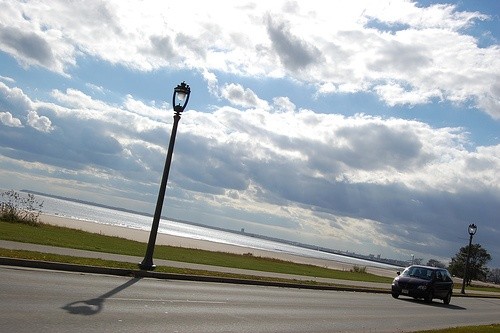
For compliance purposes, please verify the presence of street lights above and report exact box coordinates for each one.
[459,223,477,294]
[139,82,191,271]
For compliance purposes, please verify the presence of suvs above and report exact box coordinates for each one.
[391,265,454,305]
